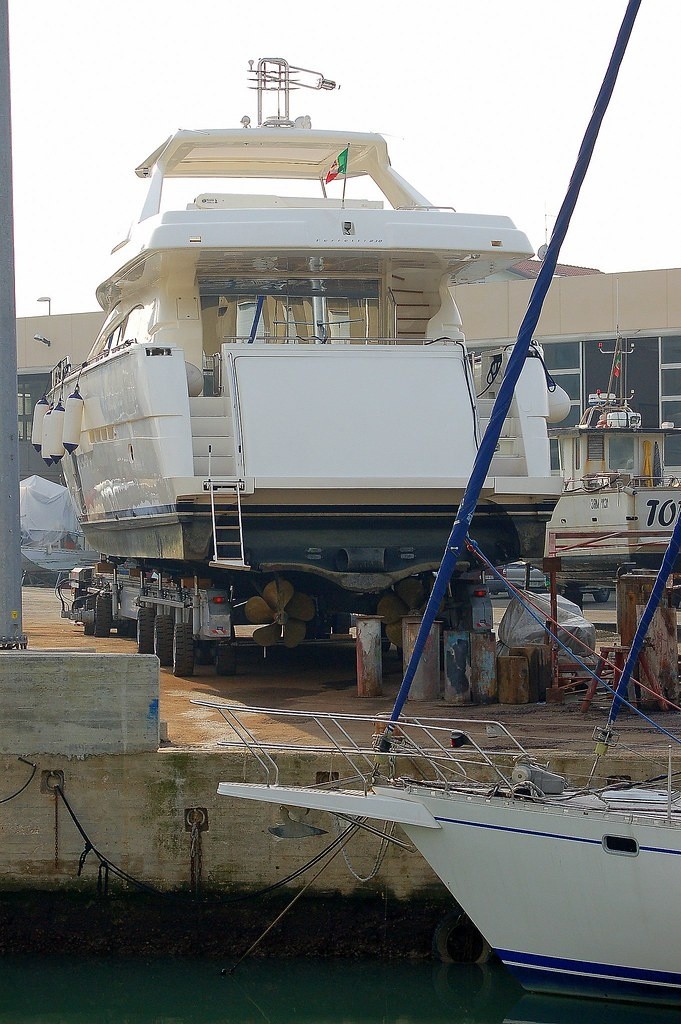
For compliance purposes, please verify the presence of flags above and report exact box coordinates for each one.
[326,148,348,184]
[612,351,622,377]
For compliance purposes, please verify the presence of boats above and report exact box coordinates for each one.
[27,53,573,582]
